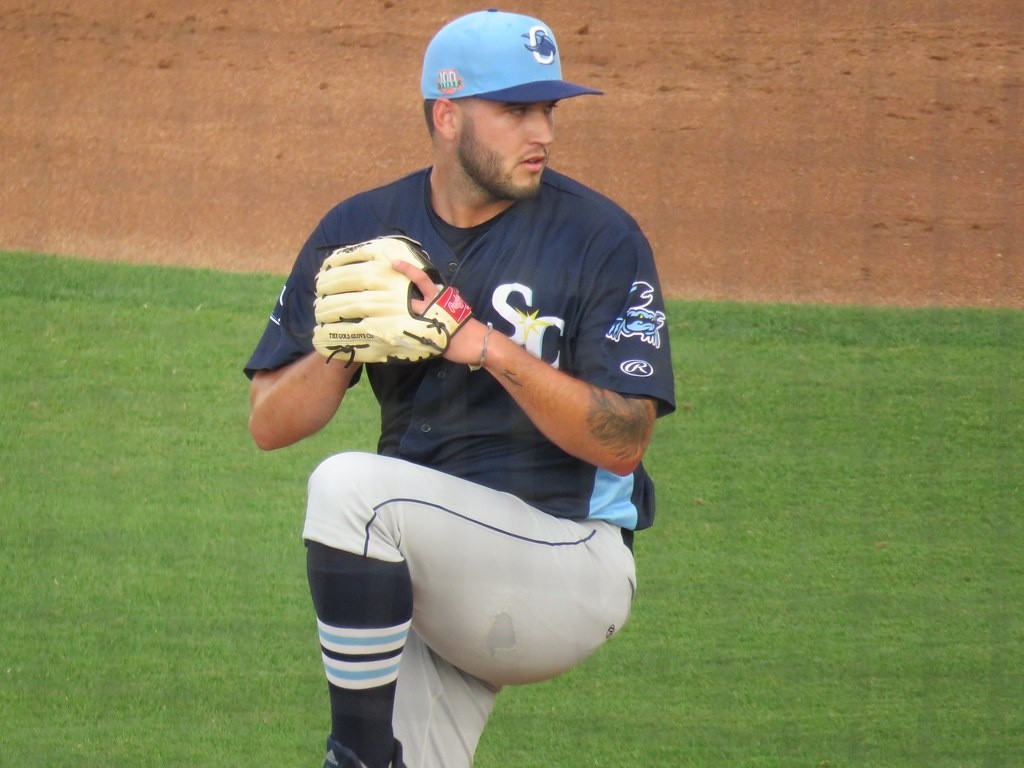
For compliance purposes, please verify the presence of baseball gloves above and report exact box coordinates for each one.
[310,235,478,367]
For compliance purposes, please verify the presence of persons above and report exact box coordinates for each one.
[242,9,678,768]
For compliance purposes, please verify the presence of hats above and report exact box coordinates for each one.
[420,8,606,103]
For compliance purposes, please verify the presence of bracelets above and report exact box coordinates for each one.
[469,321,492,371]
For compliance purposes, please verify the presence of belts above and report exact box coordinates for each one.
[621,527,634,555]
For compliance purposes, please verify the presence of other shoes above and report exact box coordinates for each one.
[322,735,407,768]
